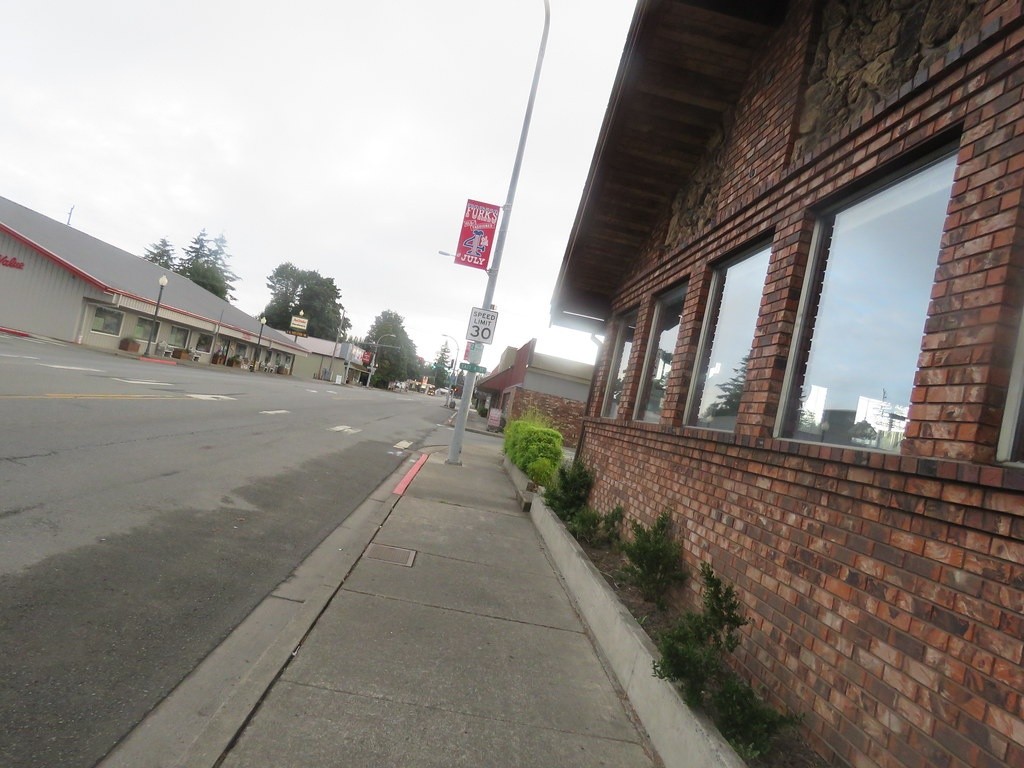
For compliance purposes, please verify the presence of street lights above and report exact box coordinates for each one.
[439,250,499,465]
[294,309,304,342]
[443,334,460,408]
[142,275,168,356]
[248,316,267,370]
[366,334,396,389]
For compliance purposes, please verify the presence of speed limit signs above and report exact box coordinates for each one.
[465,306,498,344]
